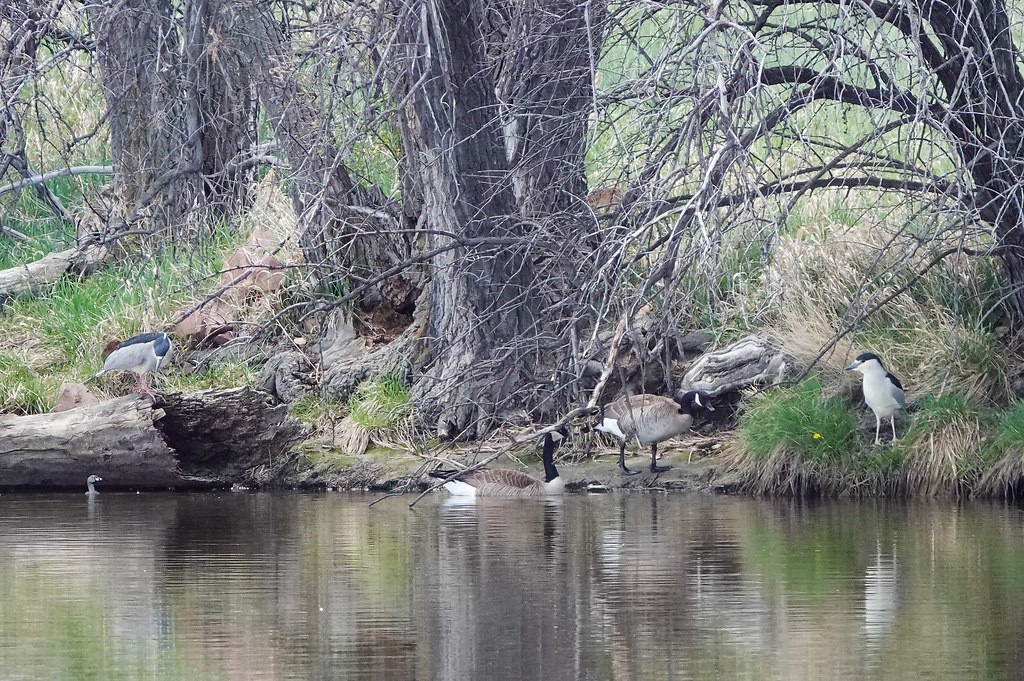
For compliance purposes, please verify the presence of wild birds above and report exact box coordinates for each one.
[76,332,178,396]
[844,352,911,446]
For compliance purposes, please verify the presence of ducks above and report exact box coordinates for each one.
[591,390,721,475]
[84,474,103,495]
[430,419,583,504]
